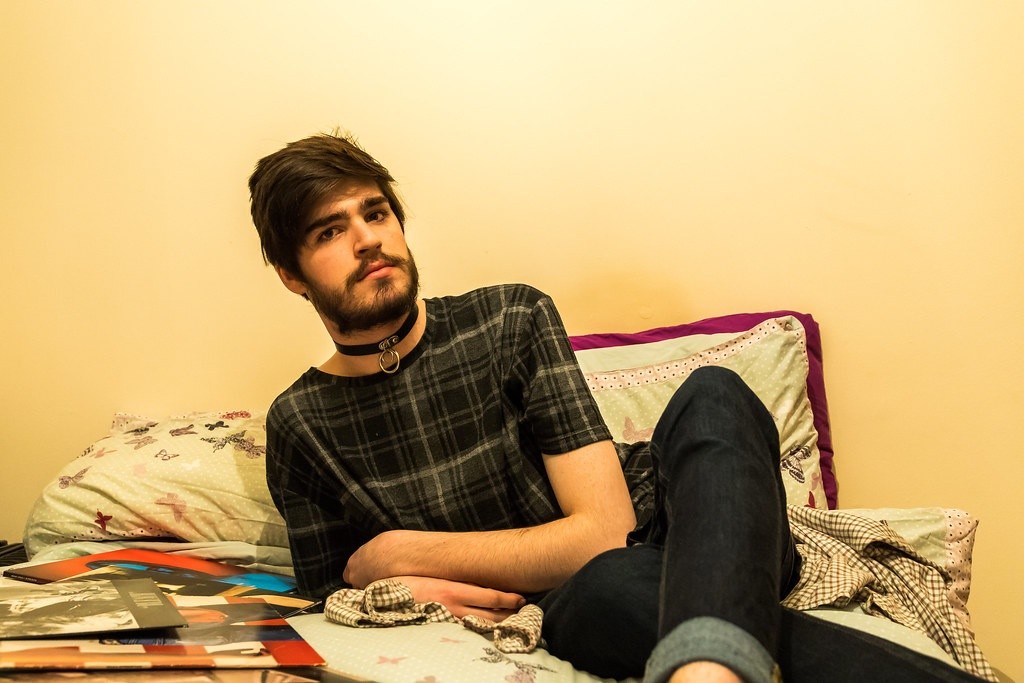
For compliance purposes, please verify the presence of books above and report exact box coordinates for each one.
[0,546,327,683]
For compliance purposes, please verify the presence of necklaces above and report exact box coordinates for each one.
[333,301,418,374]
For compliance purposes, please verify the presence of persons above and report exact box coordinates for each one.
[250,134,988,683]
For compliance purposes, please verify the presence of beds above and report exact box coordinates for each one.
[0,408,1008,683]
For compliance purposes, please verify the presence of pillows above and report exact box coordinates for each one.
[568,311,839,511]
[582,314,831,510]
